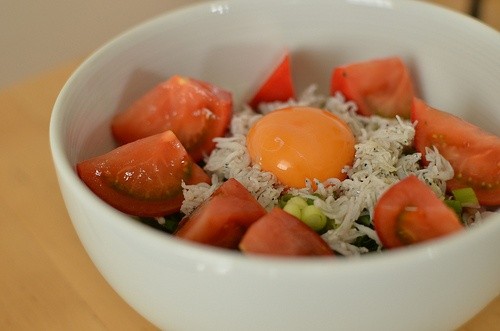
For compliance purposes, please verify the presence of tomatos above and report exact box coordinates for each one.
[76,44,500,258]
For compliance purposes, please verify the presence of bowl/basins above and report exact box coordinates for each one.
[47,1,499,331]
